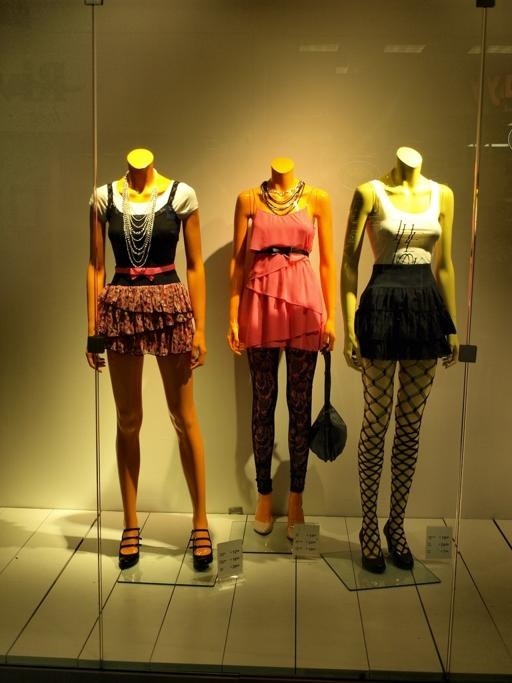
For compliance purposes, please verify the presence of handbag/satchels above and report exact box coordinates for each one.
[309,403,347,463]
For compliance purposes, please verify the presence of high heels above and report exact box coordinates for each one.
[359,528,386,574]
[189,529,213,565]
[287,524,304,540]
[383,522,414,569]
[119,528,142,569]
[253,516,273,535]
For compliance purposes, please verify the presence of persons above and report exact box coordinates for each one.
[224,157,336,539]
[84,148,214,570]
[340,145,459,573]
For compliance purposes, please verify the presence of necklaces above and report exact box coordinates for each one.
[123,173,157,270]
[260,179,305,215]
